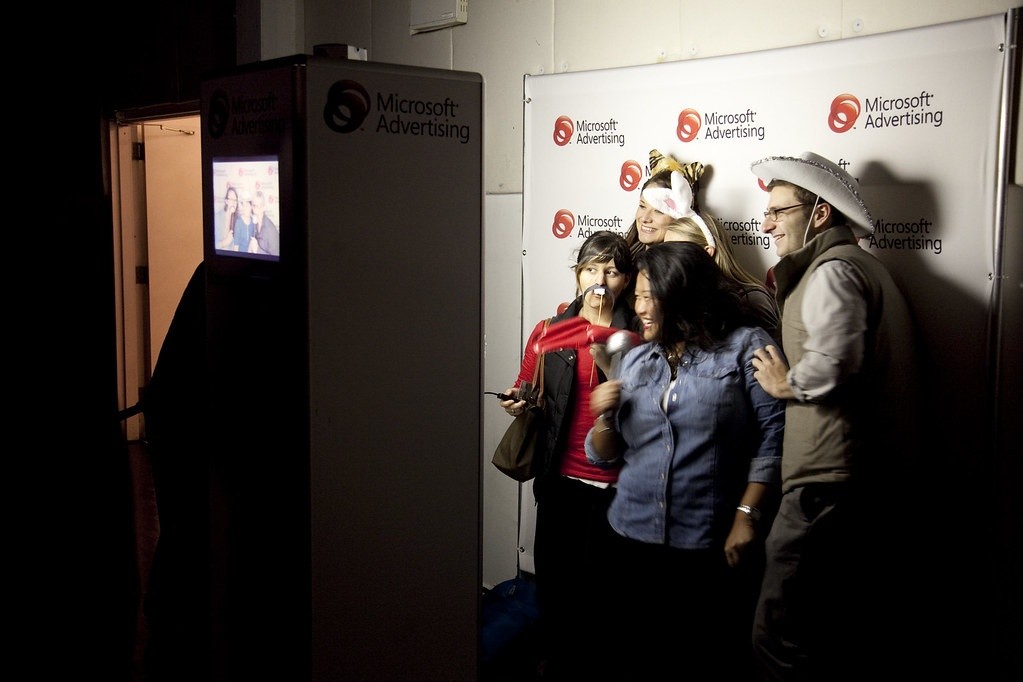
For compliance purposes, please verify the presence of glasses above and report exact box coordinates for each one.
[764,198,823,223]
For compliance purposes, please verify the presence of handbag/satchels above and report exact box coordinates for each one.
[490,316,554,484]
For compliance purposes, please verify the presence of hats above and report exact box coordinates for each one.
[752,150,875,242]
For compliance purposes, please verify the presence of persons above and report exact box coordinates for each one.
[584,241,789,680]
[749,152,916,682]
[499,230,649,680]
[214,188,279,255]
[663,213,782,350]
[623,166,700,267]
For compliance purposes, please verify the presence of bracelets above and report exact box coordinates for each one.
[736,504,762,521]
[592,414,616,435]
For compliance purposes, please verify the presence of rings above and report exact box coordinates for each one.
[511,410,515,413]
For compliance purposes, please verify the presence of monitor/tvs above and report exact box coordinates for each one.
[213,155,280,261]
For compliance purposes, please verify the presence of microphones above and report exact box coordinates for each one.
[603,329,631,430]
